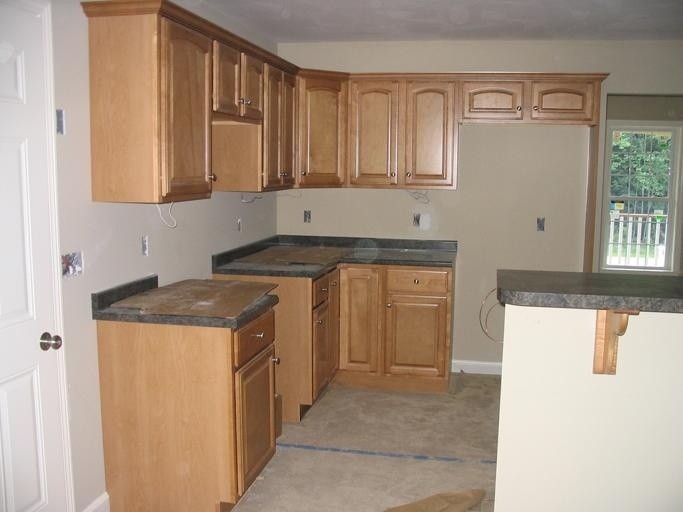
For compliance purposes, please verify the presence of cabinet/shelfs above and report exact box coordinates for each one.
[383,261,457,393]
[299,66,348,190]
[457,70,612,126]
[90,307,280,511]
[337,260,389,391]
[212,266,329,425]
[328,266,342,384]
[348,71,458,190]
